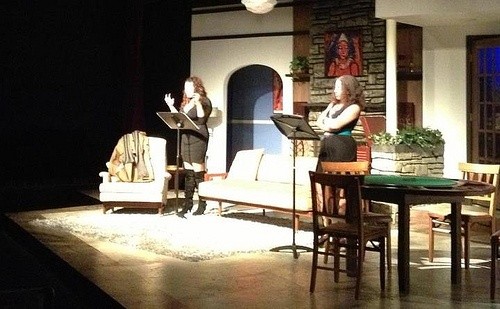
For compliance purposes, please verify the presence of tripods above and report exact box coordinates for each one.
[269,114,320,260]
[156,111,200,218]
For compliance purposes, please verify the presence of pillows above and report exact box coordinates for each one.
[225,148,265,182]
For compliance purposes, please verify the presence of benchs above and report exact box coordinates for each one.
[198,154,317,231]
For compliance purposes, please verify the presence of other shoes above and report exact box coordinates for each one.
[316,235,328,248]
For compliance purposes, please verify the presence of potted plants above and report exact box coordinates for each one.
[291,56,310,74]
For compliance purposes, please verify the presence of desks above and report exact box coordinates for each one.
[332,174,496,296]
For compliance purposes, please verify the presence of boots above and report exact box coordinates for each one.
[193,171,207,215]
[180,169,195,214]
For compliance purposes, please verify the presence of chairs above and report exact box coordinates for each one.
[309,159,389,295]
[426,162,499,270]
[98,133,174,215]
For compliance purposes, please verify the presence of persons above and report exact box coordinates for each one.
[164,77,212,215]
[314,76,366,252]
[327,33,358,76]
